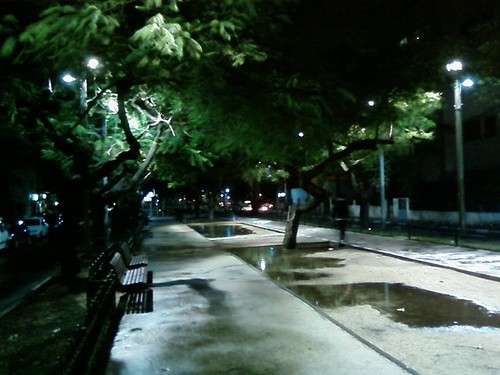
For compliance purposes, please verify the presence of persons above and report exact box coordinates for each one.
[334,203,351,248]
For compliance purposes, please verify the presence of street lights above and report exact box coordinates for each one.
[368,101,390,225]
[445,61,473,228]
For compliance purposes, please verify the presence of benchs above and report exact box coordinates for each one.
[141,224,150,232]
[109,239,151,302]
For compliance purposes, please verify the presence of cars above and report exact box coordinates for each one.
[4,217,33,247]
[0,217,9,249]
[45,213,63,229]
[22,216,49,237]
